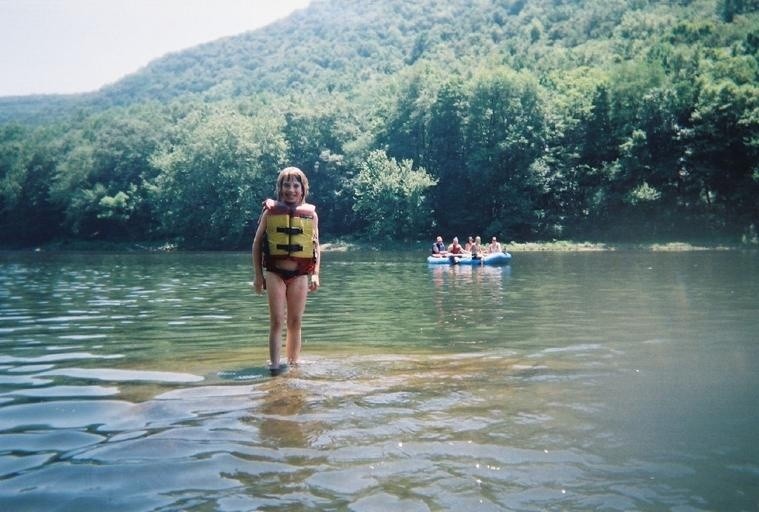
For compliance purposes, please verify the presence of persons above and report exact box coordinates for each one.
[431,236,503,258]
[252,166,321,370]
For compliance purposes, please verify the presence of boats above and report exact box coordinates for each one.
[426,253,513,267]
